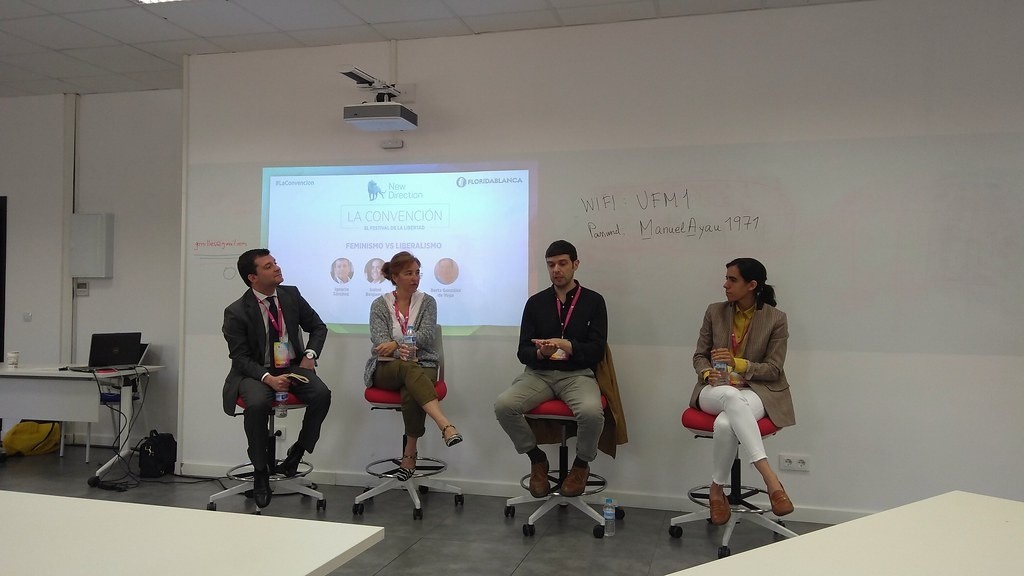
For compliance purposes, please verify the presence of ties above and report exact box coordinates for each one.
[264,296,280,371]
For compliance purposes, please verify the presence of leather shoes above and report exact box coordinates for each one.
[281,442,305,476]
[559,461,591,497]
[253,469,272,509]
[529,457,550,498]
[767,482,795,517]
[708,491,731,526]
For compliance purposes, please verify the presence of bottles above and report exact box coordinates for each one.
[602,498,615,537]
[275,391,288,419]
[402,325,416,362]
[712,360,728,385]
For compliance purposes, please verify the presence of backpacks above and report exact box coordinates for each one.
[138,429,177,478]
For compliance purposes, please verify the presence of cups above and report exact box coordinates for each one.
[6,351,24,368]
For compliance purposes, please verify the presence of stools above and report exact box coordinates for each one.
[206,328,325,511]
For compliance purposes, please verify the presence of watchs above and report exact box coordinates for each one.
[540,350,550,359]
[304,352,315,359]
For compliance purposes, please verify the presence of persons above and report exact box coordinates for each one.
[689,258,797,525]
[364,258,383,284]
[222,249,332,508]
[494,240,608,498]
[333,258,351,284]
[363,251,463,481]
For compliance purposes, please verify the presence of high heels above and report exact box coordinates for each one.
[396,448,418,481]
[441,424,464,448]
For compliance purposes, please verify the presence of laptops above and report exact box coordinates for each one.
[67,332,150,371]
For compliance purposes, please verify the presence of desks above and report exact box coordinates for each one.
[0,365,164,487]
[0,491,386,576]
[661,488,1024,576]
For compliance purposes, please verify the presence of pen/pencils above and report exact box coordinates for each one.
[587,321,590,325]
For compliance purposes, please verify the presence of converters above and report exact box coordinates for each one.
[97,482,119,490]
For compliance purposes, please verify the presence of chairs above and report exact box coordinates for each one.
[353,324,464,519]
[505,340,625,538]
[669,334,799,557]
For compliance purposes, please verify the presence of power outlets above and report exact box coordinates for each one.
[780,453,811,471]
[273,427,285,441]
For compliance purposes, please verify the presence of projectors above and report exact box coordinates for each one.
[343,102,418,130]
